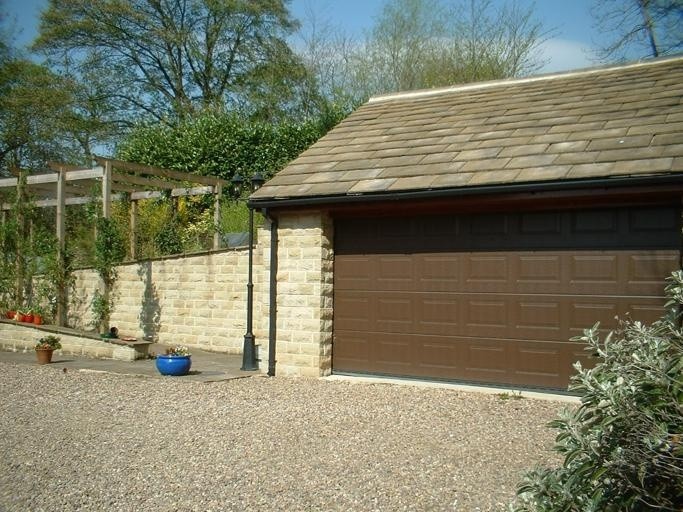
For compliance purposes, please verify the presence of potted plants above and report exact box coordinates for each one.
[34,335,62,365]
[7,304,42,325]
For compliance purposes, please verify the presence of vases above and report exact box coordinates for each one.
[156,354,190,376]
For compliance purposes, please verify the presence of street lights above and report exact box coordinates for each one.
[230,173,265,371]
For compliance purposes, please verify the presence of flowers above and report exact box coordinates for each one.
[164,346,190,356]
